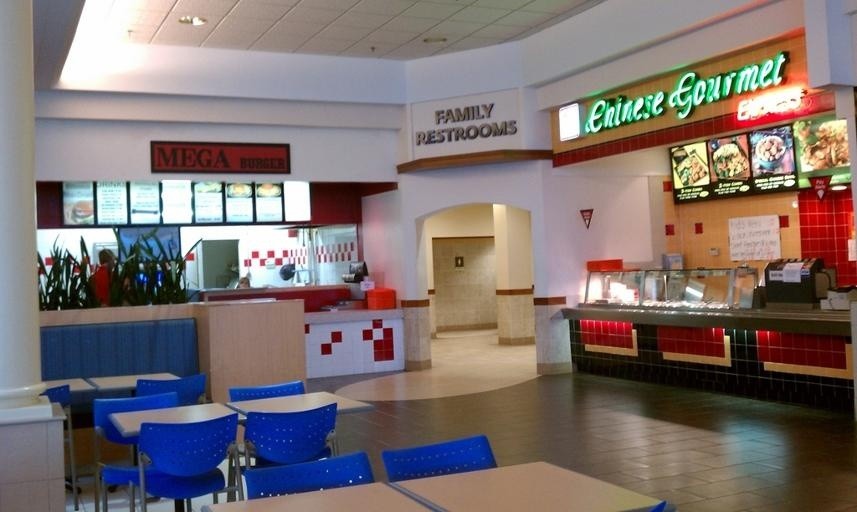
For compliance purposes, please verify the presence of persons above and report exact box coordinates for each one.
[86,250,122,307]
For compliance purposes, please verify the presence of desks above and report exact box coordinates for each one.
[40,378,95,395]
[385,461,665,512]
[207,482,434,512]
[225,391,375,416]
[89,373,183,392]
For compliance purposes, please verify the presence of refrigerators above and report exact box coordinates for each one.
[186,239,239,289]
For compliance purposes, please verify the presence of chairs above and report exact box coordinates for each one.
[383,436,497,481]
[40,385,77,500]
[244,458,375,500]
[93,375,337,501]
